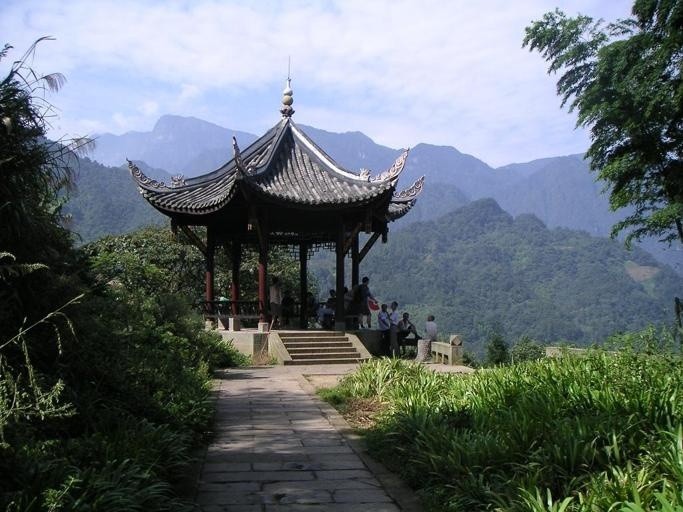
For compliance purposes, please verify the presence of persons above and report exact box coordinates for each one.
[424,314,438,341]
[377,303,391,357]
[397,312,422,355]
[354,276,377,329]
[269,277,287,330]
[280,286,354,330]
[389,302,399,358]
